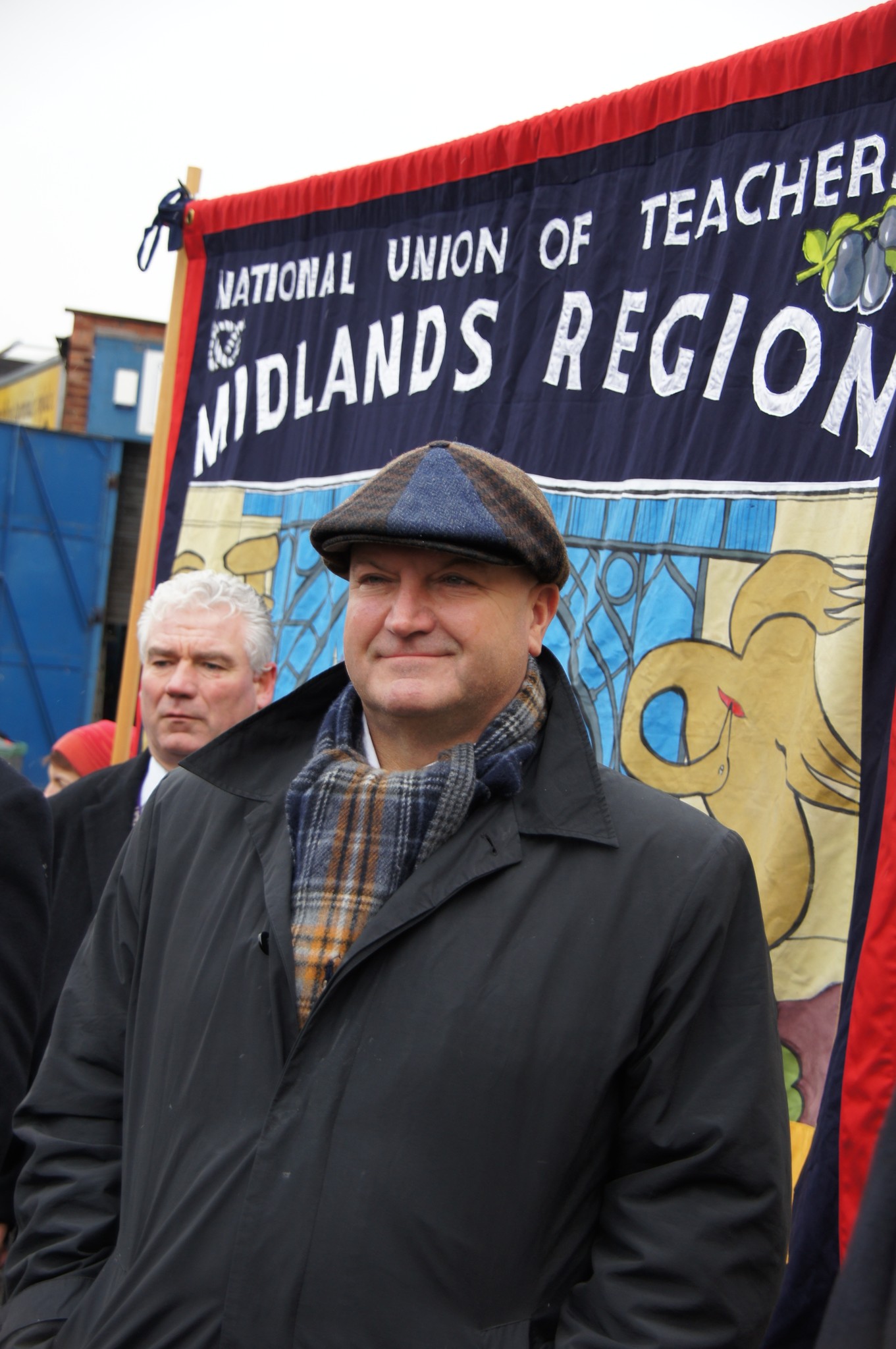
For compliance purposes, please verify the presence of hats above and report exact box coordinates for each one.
[51,720,137,778]
[312,439,568,592]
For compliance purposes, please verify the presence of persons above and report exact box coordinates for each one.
[34,719,140,801]
[42,571,281,1050]
[0,443,796,1349]
[0,753,51,1223]
